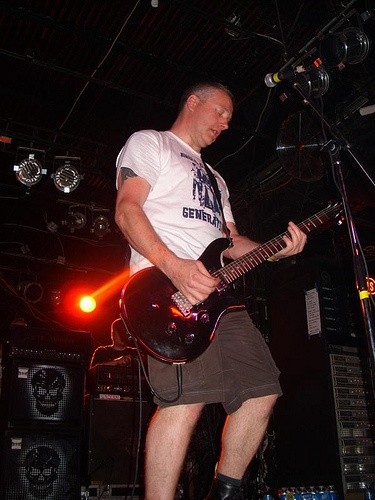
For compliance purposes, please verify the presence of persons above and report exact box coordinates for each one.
[90,319,140,368]
[113,82,307,500]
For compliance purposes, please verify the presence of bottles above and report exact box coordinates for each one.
[279,485,339,500]
[346,482,370,500]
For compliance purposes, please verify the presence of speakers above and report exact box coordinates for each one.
[76,397,153,488]
[0,359,85,434]
[0,430,81,500]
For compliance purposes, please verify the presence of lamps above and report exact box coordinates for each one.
[13,147,48,194]
[291,66,332,100]
[51,156,85,194]
[311,27,369,72]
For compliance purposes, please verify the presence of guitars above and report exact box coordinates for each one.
[116,204,346,365]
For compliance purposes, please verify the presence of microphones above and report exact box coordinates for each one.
[264,64,314,88]
